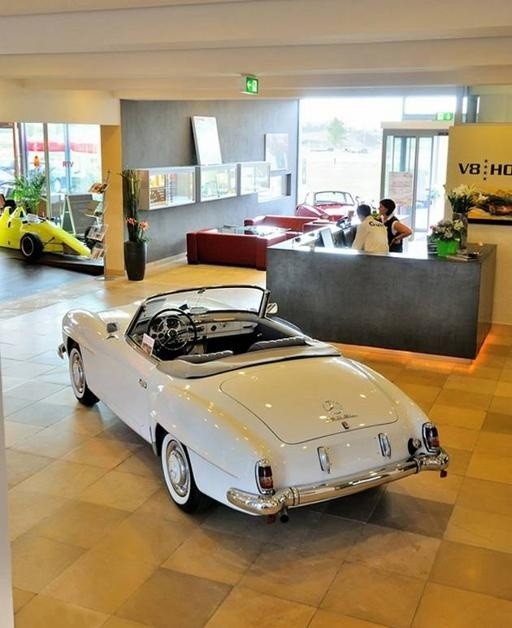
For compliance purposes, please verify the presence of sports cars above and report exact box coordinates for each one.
[56,281,454,521]
[0,194,91,258]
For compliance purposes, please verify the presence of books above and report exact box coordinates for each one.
[85,183,108,259]
[446,252,480,261]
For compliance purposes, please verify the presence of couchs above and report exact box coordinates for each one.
[186,214,335,271]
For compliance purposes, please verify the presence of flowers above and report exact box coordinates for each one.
[428,216,464,243]
[440,180,490,217]
[115,168,154,249]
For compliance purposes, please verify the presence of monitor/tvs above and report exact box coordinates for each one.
[342,225,356,247]
[320,228,334,249]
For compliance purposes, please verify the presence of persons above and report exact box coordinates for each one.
[351,204,390,252]
[378,198,411,252]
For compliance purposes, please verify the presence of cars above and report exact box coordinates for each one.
[343,145,369,154]
[0,155,96,195]
[296,190,363,223]
[370,180,442,212]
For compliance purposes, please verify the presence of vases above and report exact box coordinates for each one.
[450,212,469,249]
[121,238,149,283]
[433,237,462,257]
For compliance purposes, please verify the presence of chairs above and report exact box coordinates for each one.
[172,347,234,366]
[246,336,306,351]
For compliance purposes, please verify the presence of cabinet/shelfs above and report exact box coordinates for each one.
[82,168,119,282]
[133,159,272,215]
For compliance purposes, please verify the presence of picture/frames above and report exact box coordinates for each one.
[189,114,223,167]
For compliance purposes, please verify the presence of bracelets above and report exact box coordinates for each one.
[394,237,398,240]
